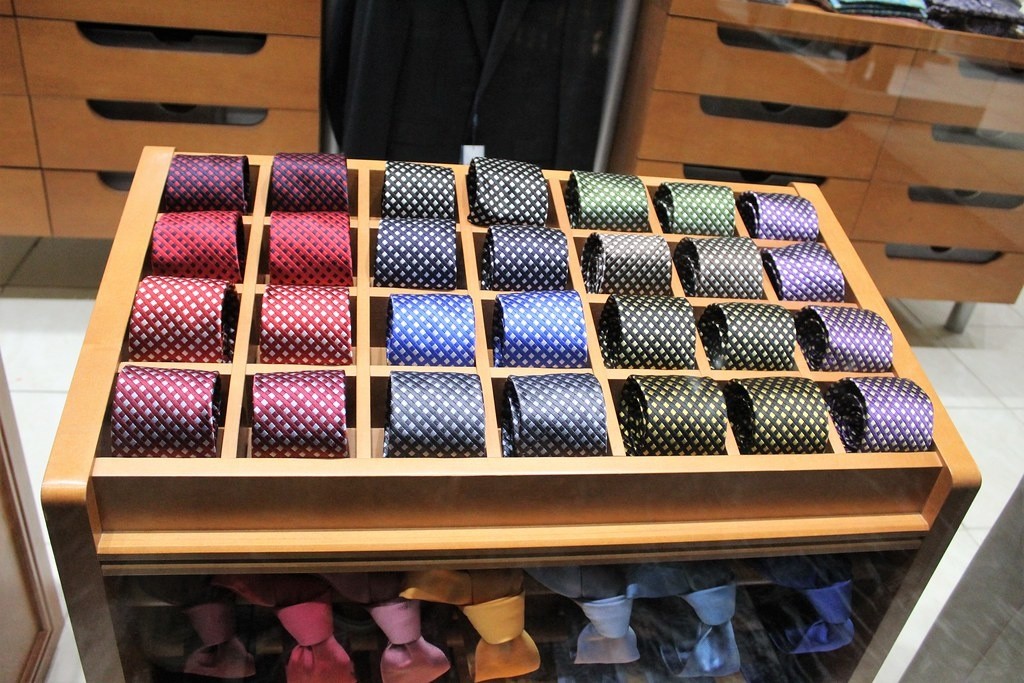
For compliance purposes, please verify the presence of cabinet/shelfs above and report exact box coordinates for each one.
[0,0,1024,683]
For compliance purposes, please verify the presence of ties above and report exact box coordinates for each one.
[110,151,932,457]
[139,555,854,683]
